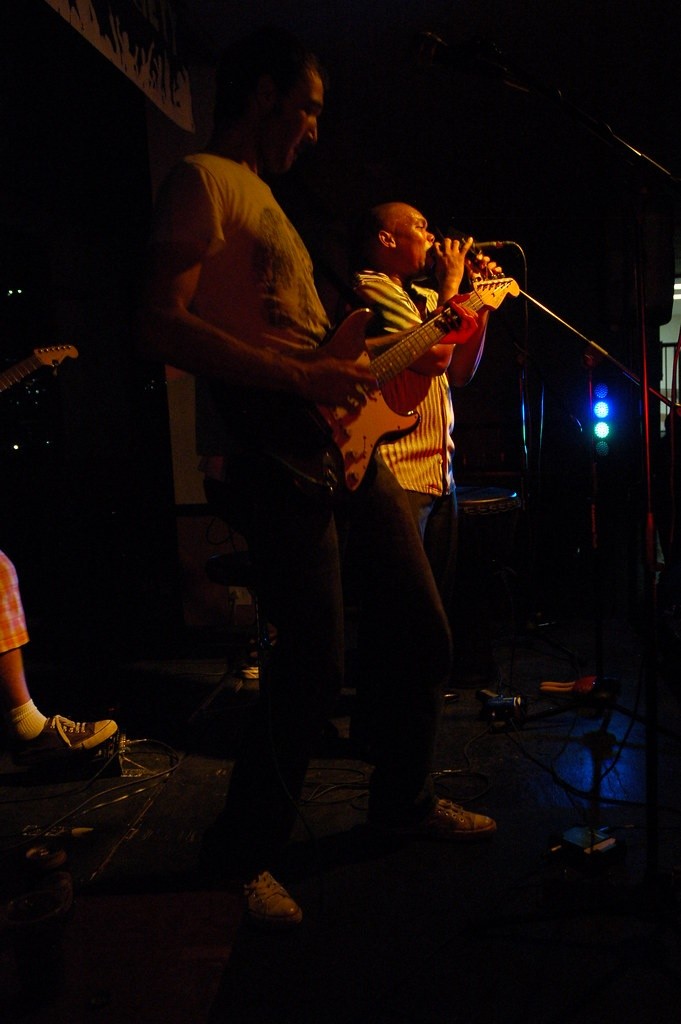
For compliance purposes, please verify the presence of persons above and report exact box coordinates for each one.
[0,550,119,766]
[347,202,505,759]
[154,34,497,930]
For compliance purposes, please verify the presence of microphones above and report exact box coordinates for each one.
[416,33,510,77]
[430,241,516,257]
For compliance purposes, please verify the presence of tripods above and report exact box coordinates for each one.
[471,251,680,822]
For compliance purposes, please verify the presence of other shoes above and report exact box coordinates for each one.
[413,768,489,805]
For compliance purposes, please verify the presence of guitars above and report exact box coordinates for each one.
[263,274,523,496]
[3,342,81,394]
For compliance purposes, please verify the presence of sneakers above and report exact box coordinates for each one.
[376,796,497,837]
[232,869,302,925]
[11,715,117,767]
